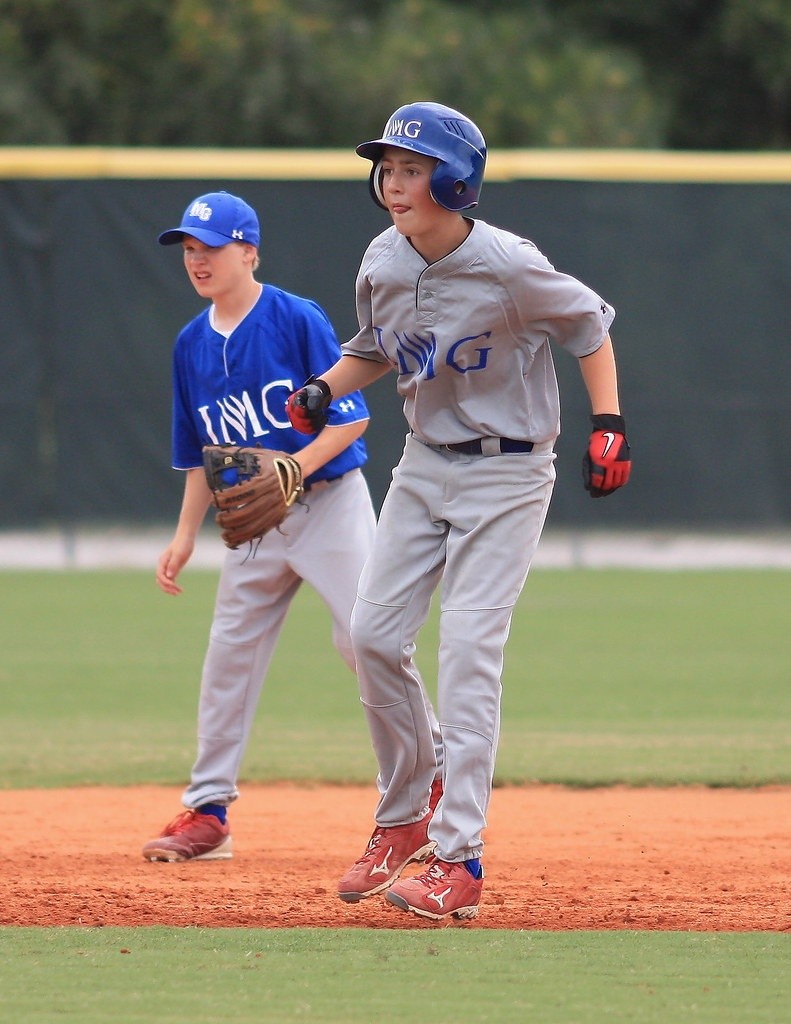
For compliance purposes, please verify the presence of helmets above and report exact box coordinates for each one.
[355,101,487,211]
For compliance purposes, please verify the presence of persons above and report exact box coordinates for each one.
[143,191,443,865]
[284,101,631,920]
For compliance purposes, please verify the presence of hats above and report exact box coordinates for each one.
[157,191,260,248]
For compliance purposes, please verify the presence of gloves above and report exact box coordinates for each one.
[285,374,333,435]
[582,412,631,498]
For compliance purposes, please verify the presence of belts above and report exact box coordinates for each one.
[305,475,343,493]
[443,435,534,454]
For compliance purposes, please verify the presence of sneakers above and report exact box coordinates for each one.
[143,808,233,862]
[428,778,444,812]
[337,809,433,905]
[385,853,484,919]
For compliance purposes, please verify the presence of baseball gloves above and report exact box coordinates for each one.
[206,441,301,550]
